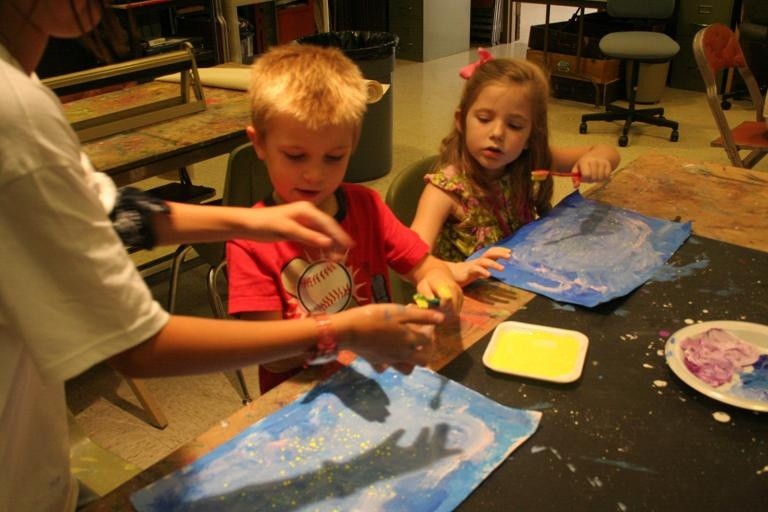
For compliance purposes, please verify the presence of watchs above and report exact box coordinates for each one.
[306,310,339,367]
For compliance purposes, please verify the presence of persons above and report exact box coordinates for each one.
[400,46,622,289]
[0,1,448,512]
[227,45,463,395]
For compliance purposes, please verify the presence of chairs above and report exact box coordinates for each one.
[579,2,768,169]
[166,142,442,404]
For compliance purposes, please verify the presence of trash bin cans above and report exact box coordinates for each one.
[297,31,399,183]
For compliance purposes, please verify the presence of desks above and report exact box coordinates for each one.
[69,152,768,511]
[58,59,254,430]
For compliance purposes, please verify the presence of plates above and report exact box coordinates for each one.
[665,319,764,414]
[481,319,589,386]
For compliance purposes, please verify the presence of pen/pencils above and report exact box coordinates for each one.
[418,296,439,305]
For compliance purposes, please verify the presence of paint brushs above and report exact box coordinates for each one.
[530,170,578,176]
